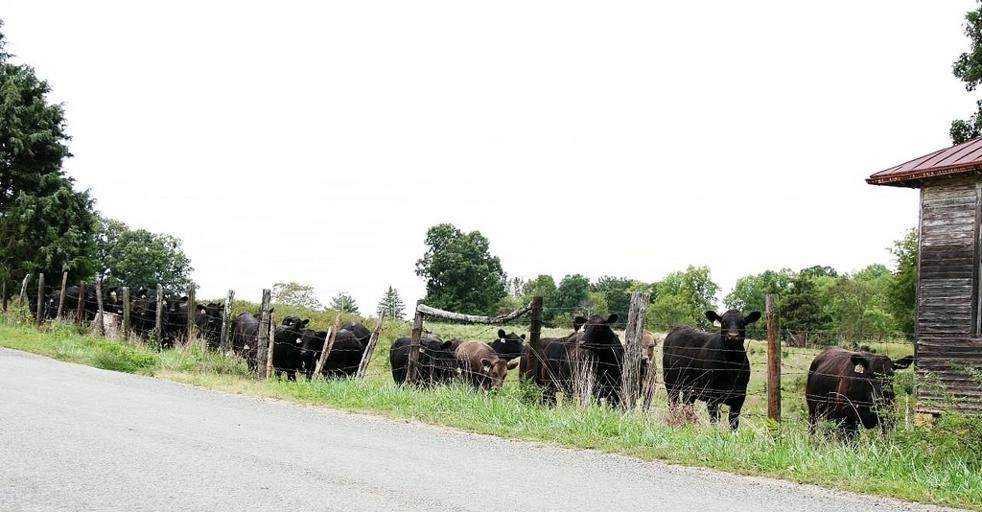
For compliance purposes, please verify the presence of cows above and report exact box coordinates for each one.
[28,277,373,379]
[660,307,761,431]
[388,312,664,412]
[804,345,914,444]
